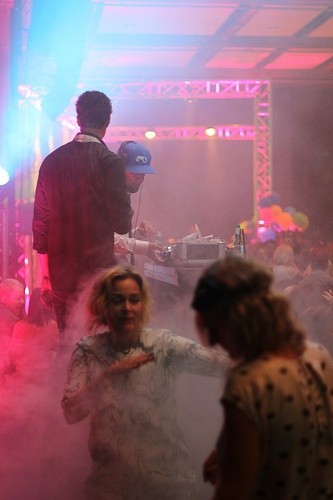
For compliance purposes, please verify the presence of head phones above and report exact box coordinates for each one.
[119,140,136,163]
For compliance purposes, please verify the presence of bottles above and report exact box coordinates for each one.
[232,224,244,257]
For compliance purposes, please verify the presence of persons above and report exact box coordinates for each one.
[190,254,333,500]
[0,236,333,429]
[113,140,170,263]
[61,264,236,500]
[31,90,134,347]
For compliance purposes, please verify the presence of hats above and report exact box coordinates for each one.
[116,140,157,174]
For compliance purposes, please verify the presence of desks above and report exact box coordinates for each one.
[140,261,206,316]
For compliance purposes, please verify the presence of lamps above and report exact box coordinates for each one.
[144,127,157,141]
[204,126,217,136]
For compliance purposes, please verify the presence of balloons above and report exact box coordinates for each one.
[250,192,310,244]
